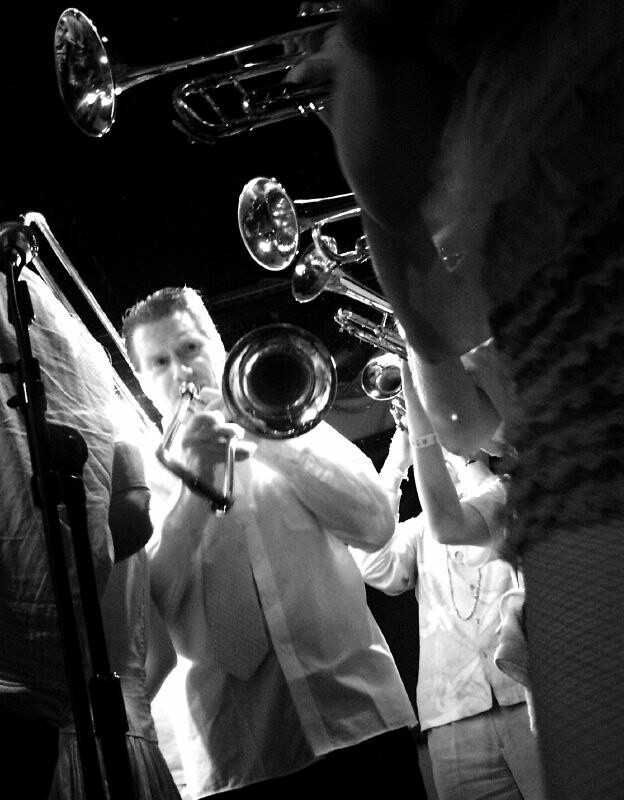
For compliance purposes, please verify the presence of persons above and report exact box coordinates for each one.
[0,418,183,800]
[123,283,429,800]
[329,0,624,800]
[345,361,546,800]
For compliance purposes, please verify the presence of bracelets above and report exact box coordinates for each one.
[406,433,438,452]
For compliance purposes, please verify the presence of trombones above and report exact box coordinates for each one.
[157,324,341,516]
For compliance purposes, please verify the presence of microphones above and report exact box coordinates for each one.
[0,221,38,273]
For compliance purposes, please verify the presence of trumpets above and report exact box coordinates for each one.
[236,174,374,273]
[361,350,408,435]
[54,6,344,141]
[291,234,412,364]
